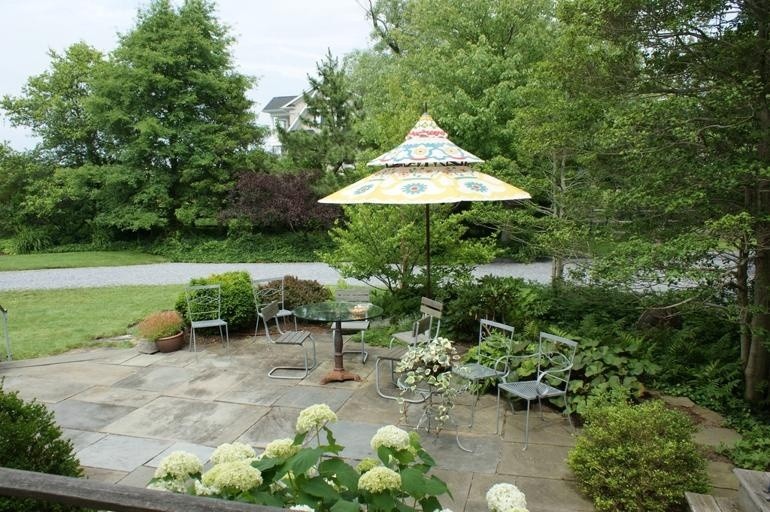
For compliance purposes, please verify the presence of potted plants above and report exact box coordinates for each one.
[395,334,461,443]
[138,309,183,353]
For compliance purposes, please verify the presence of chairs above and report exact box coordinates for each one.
[451,318,515,432]
[371,316,435,404]
[330,288,373,365]
[496,330,577,452]
[182,283,232,361]
[389,296,443,350]
[260,300,317,381]
[248,273,298,336]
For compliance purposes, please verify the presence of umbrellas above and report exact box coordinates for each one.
[317,112,532,298]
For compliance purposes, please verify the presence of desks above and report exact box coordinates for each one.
[288,301,384,386]
[395,370,473,455]
